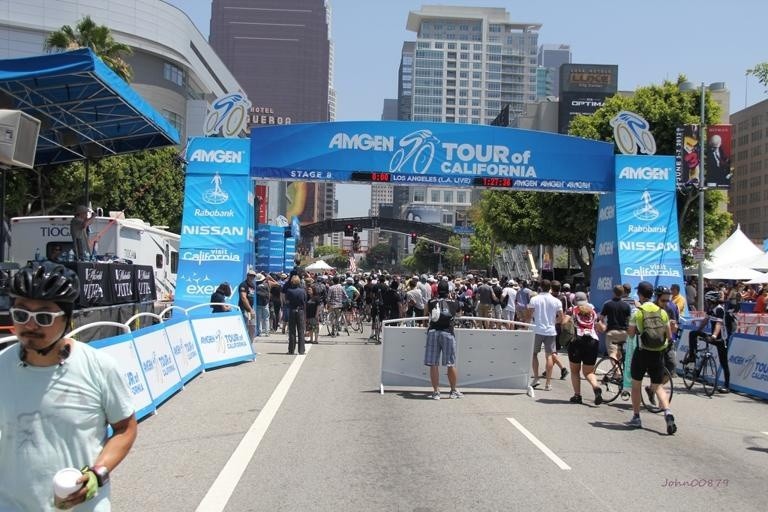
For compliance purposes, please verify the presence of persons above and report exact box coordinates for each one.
[210,282,231,311]
[627,281,678,434]
[686,280,697,304]
[424,279,463,400]
[542,280,569,379]
[707,135,730,182]
[718,283,768,313]
[599,283,636,378]
[47,243,60,261]
[645,285,680,405]
[669,283,685,312]
[285,275,307,355]
[561,283,575,308]
[526,279,564,391]
[70,206,98,260]
[562,292,606,406]
[0,262,137,512]
[682,290,732,393]
[239,270,541,344]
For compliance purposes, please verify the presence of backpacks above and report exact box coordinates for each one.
[636,306,666,348]
[430,300,453,330]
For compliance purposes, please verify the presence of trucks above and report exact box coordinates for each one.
[7,209,181,307]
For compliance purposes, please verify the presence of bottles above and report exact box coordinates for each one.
[67,249,75,261]
[34,248,41,260]
[91,241,99,256]
[695,352,701,369]
[673,328,681,342]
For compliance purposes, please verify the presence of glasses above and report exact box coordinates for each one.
[9,307,65,327]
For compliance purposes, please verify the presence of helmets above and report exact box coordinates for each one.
[655,286,670,295]
[704,291,720,302]
[8,260,80,303]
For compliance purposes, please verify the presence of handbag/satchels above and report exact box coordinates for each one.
[501,296,509,309]
[725,312,738,334]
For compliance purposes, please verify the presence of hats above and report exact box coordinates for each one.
[488,278,499,285]
[255,273,265,282]
[247,270,256,275]
[438,281,448,294]
[73,204,89,215]
[634,281,654,293]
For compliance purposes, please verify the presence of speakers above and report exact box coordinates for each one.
[0,108,42,170]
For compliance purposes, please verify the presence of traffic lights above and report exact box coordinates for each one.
[412,231,417,243]
[464,254,470,266]
[345,224,353,236]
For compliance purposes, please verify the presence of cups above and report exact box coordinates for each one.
[53,466,84,511]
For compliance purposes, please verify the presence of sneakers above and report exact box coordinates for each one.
[449,390,464,398]
[624,386,677,435]
[683,356,695,362]
[594,388,602,405]
[531,367,568,391]
[432,391,440,400]
[570,395,582,404]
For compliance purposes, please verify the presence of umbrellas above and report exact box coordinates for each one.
[682,223,768,286]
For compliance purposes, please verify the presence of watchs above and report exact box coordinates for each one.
[88,465,111,485]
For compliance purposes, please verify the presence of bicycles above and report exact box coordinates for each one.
[593,338,673,414]
[683,332,719,396]
[304,296,383,346]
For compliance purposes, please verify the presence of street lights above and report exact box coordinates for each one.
[678,81,728,314]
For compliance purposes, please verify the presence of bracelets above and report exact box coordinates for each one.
[712,335,716,337]
